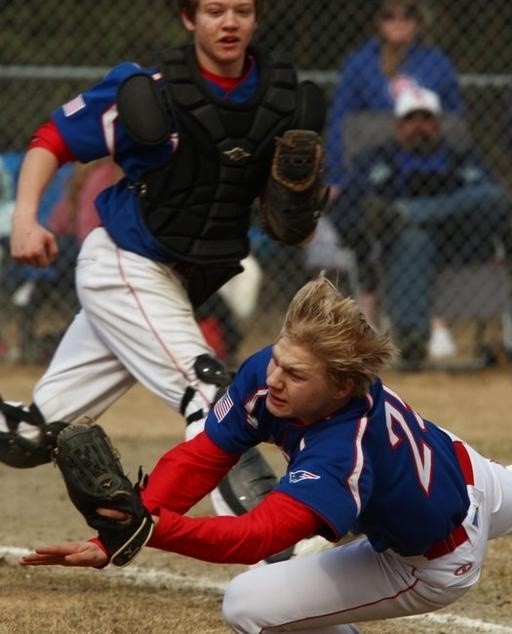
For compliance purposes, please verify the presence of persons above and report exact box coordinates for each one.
[340,84,510,373]
[11,151,138,369]
[317,0,474,341]
[1,0,324,563]
[16,269,512,631]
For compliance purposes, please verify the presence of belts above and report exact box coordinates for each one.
[426,441,472,559]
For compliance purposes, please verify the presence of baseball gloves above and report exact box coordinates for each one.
[52,423,154,567]
[261,129,328,246]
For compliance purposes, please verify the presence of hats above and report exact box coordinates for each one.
[394,87,440,118]
